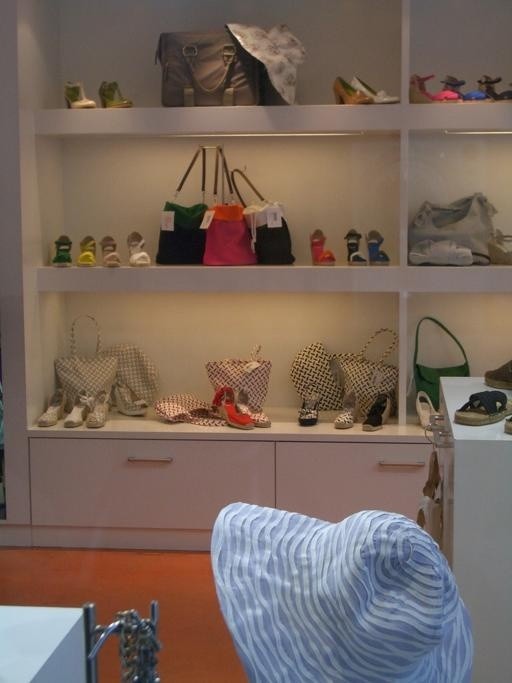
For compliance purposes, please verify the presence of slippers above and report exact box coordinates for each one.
[454,391,511,426]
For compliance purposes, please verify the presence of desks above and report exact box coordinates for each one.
[0,605,87,683]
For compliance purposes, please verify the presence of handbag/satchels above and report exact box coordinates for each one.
[288,342,343,409]
[202,146,258,265]
[159,31,264,106]
[409,192,493,266]
[102,344,159,407]
[232,169,295,264]
[337,358,399,419]
[204,359,271,412]
[416,495,441,542]
[54,353,118,413]
[414,363,469,412]
[156,148,208,264]
[154,393,228,426]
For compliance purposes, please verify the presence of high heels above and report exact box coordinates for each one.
[344,229,367,266]
[311,229,336,265]
[365,230,390,266]
[64,81,96,108]
[64,389,92,428]
[101,236,121,267]
[477,77,511,101]
[86,390,110,428]
[213,384,252,429]
[411,75,460,102]
[52,236,72,267]
[38,389,66,426]
[299,382,319,425]
[333,76,374,104]
[99,82,132,108]
[127,232,150,266]
[114,385,148,416]
[334,389,360,429]
[78,236,96,266]
[442,76,489,102]
[362,394,391,431]
[236,387,271,427]
[416,391,438,431]
[352,76,400,103]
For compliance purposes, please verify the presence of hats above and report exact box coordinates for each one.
[225,21,309,105]
[210,501,474,682]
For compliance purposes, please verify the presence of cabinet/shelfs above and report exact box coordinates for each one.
[0,0,512,552]
[432,377,512,683]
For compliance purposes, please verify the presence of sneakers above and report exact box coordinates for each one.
[485,360,511,390]
